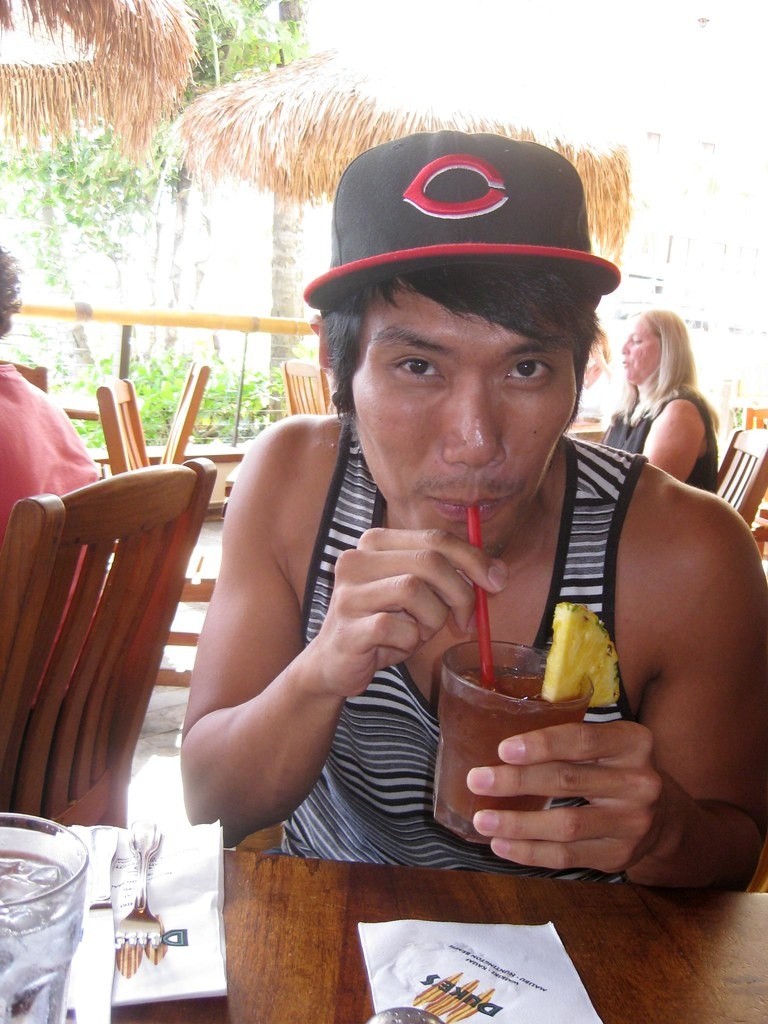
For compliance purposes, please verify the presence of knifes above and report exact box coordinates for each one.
[81,827,116,1024]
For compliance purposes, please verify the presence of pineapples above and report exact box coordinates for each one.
[541,602,620,708]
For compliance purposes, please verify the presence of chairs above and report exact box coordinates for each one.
[1,357,335,839]
[718,402,768,566]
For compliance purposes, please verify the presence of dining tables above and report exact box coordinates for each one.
[112,847,768,1023]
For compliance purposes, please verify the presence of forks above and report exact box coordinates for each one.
[113,820,165,952]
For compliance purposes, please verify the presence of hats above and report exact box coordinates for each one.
[303,130,621,317]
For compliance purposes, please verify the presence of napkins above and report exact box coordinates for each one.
[357,920,605,1023]
[69,821,228,1006]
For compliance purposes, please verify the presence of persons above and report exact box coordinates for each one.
[601,309,719,496]
[1,245,101,836]
[178,130,768,891]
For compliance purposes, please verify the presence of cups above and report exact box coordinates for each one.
[0,813,90,1024]
[433,639,595,848]
